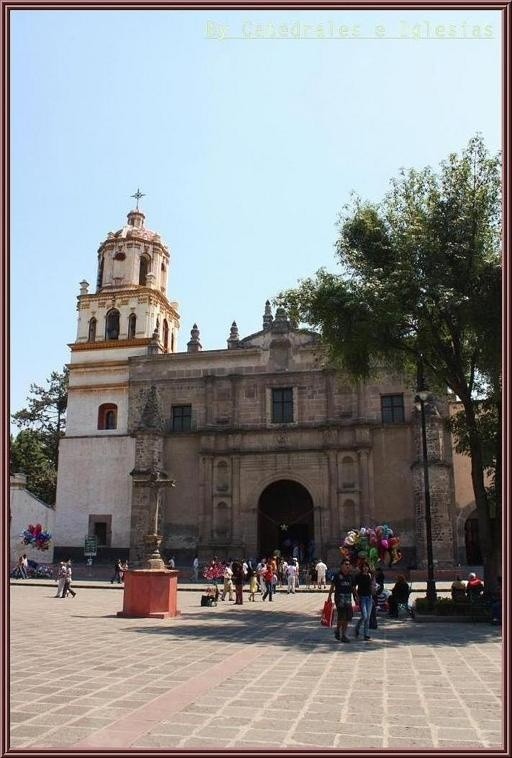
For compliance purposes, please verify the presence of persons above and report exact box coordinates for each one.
[15,554,77,599]
[491,574,502,626]
[87,555,94,576]
[451,574,466,601]
[168,558,176,570]
[466,572,484,602]
[110,558,130,584]
[188,540,411,643]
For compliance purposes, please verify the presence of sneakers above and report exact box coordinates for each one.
[334,630,373,642]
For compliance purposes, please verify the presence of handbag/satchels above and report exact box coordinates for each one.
[320,597,336,629]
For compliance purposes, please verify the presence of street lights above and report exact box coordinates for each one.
[411,370,436,581]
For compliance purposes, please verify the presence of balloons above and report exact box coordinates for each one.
[18,523,54,552]
[336,523,405,572]
